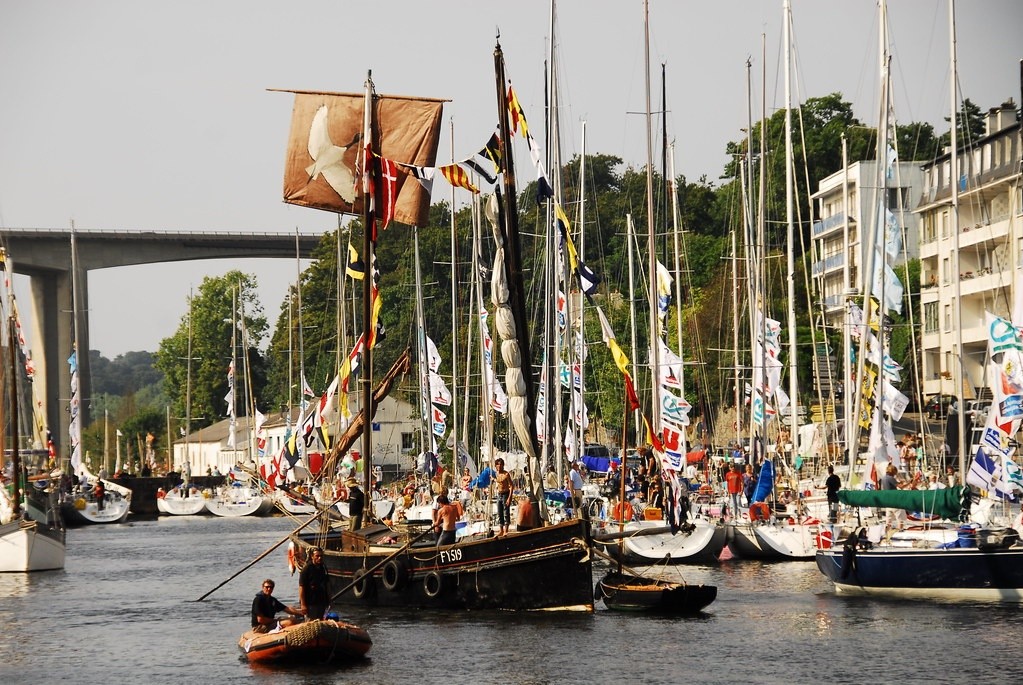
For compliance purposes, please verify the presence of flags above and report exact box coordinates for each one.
[965,310,1023,500]
[224,336,237,451]
[254,408,267,458]
[0,247,43,431]
[260,79,909,536]
[66,351,82,475]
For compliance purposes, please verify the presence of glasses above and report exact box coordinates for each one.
[263,586,274,589]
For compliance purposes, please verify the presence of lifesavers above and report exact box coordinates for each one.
[749,503,769,521]
[614,502,632,521]
[382,560,403,590]
[75,499,85,509]
[157,490,165,499]
[424,571,444,597]
[404,485,415,494]
[700,485,712,495]
[337,489,347,500]
[353,570,372,599]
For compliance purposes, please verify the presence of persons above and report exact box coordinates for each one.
[946,465,959,479]
[346,447,664,548]
[251,579,292,628]
[99,465,106,479]
[725,462,744,516]
[96,481,105,511]
[116,463,151,478]
[177,461,191,487]
[815,465,841,523]
[206,464,222,476]
[896,429,928,472]
[742,464,758,507]
[687,463,703,484]
[881,464,906,532]
[288,547,330,622]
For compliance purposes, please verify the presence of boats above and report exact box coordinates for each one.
[239,611,373,673]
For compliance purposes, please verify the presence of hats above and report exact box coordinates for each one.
[611,462,618,468]
[345,477,358,487]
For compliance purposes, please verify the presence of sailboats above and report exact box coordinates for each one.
[157,3,1023,635]
[0,247,71,576]
[48,243,134,527]
